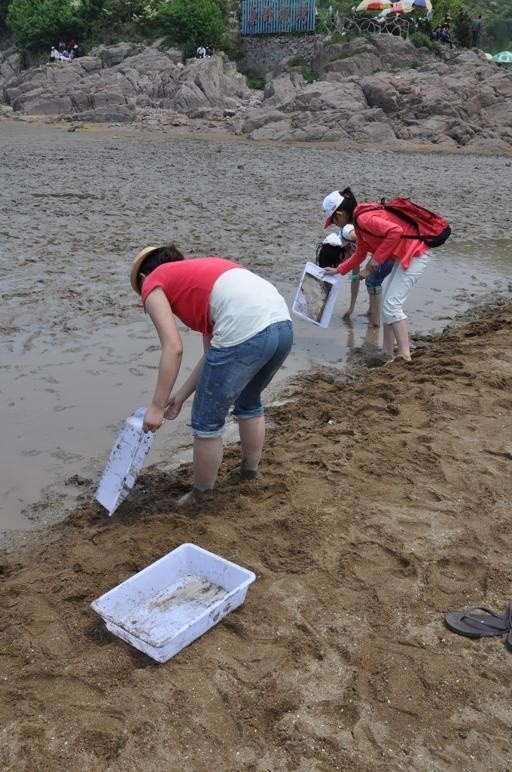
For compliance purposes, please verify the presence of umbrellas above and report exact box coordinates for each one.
[356,0,433,19]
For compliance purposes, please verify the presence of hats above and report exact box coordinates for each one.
[322,190,349,229]
[129,246,156,295]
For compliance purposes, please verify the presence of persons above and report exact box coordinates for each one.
[321,186,432,366]
[49,39,81,62]
[315,223,394,327]
[196,38,216,59]
[129,241,294,508]
[432,7,483,50]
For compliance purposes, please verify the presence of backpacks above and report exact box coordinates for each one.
[353,197,451,247]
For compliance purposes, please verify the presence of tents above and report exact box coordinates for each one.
[491,50,512,64]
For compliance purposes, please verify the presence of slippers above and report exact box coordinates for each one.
[444,603,511,647]
